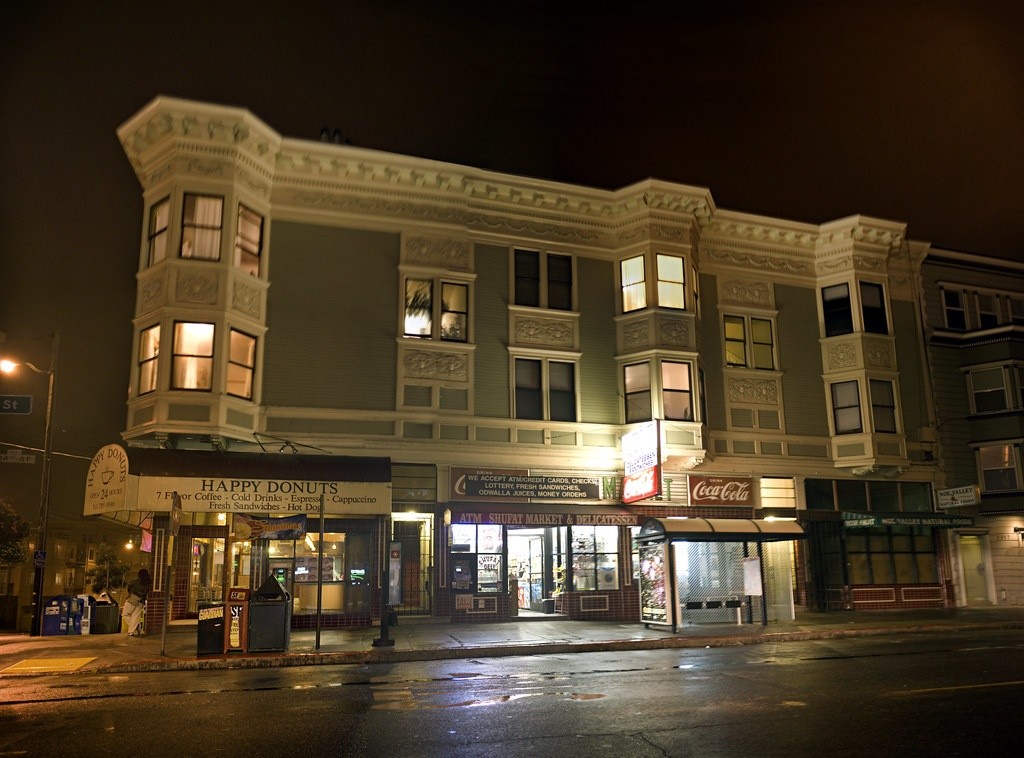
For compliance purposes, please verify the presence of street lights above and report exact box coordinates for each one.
[0,329,59,636]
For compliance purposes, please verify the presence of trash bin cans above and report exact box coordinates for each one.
[44,593,95,635]
[197,574,293,655]
[94,591,120,634]
